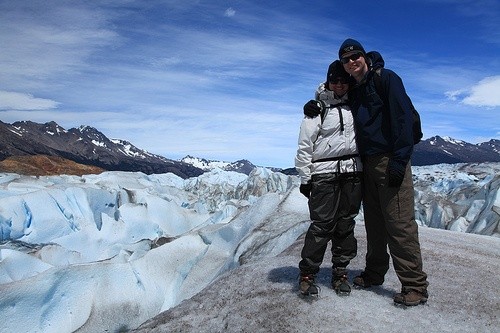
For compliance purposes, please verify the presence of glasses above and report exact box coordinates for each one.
[340,52,362,64]
[331,78,349,84]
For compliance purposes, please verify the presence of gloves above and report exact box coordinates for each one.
[387,160,406,187]
[300,182,312,198]
[304,100,321,117]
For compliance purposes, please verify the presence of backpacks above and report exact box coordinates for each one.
[374,67,424,145]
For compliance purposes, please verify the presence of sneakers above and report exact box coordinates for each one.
[332,267,352,296]
[354,271,384,288]
[393,286,429,307]
[299,273,321,298]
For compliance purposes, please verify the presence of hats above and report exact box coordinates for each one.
[339,39,367,61]
[327,60,350,83]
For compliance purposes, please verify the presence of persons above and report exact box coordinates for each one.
[303,39,429,307]
[295,60,364,300]
